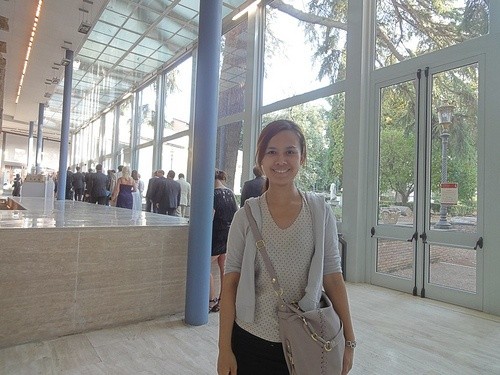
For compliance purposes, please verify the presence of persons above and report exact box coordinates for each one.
[66,164,144,211]
[217,119,358,375]
[52,171,59,192]
[13,174,22,196]
[209,167,267,311]
[146,170,191,218]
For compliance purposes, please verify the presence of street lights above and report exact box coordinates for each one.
[435,99,456,229]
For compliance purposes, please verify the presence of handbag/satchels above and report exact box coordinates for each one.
[277,290,345,375]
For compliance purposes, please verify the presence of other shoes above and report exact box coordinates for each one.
[209,297,218,313]
[212,297,221,312]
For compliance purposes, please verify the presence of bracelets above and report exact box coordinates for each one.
[346,340,356,348]
